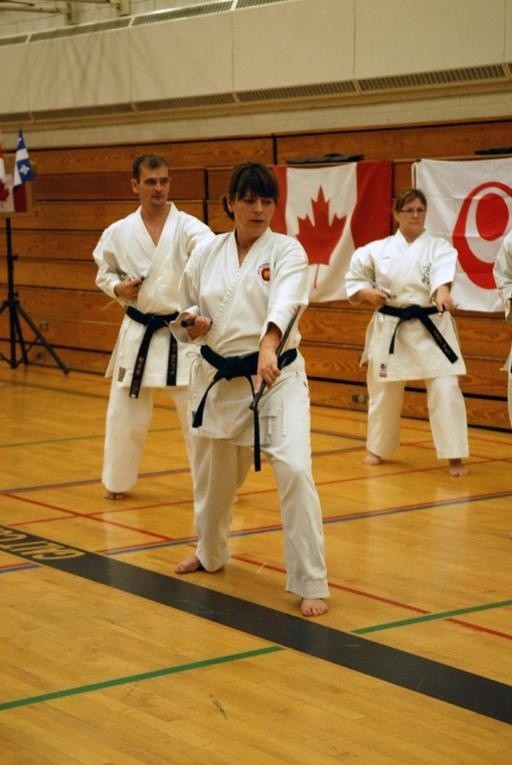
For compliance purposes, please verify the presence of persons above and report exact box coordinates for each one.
[491,229,512,428]
[171,162,331,618]
[90,152,215,501]
[344,186,472,476]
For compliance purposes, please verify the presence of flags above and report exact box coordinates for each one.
[12,130,34,213]
[411,158,511,314]
[269,159,396,305]
[1,144,15,213]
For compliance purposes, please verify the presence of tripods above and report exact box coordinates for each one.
[0,218,70,374]
